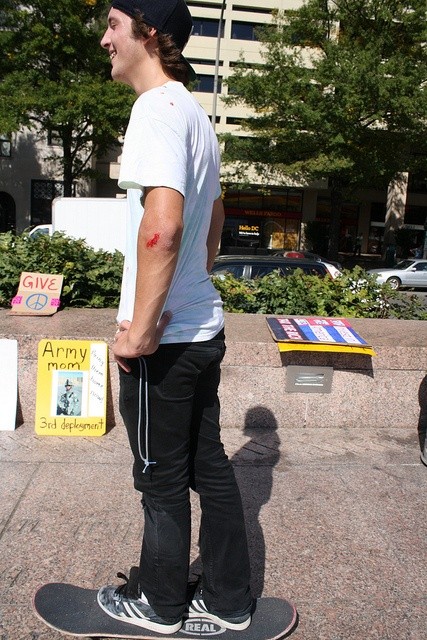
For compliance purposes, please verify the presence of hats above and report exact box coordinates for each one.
[110,1,197,81]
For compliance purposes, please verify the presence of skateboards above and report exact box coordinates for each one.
[32,582,296,640]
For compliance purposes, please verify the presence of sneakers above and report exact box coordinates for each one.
[183,573,251,631]
[97,566,182,635]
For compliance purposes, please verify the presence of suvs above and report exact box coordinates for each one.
[208,256,340,316]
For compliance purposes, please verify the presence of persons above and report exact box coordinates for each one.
[97,0,254,634]
[56,379,79,416]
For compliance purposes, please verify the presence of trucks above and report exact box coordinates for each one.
[10,197,132,259]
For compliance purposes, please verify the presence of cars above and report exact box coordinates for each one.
[315,260,389,311]
[365,258,427,292]
[267,249,343,274]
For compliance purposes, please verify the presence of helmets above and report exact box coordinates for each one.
[65,379,74,386]
